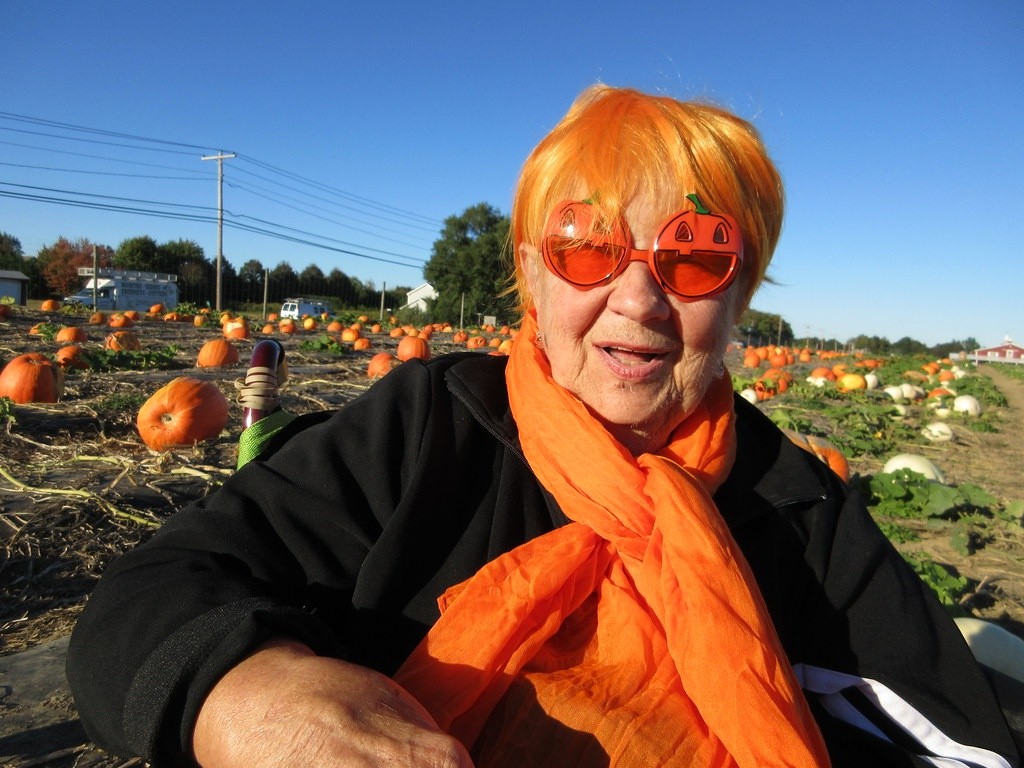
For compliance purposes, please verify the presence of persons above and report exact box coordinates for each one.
[65,79,1021,768]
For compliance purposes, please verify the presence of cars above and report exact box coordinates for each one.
[731,338,748,350]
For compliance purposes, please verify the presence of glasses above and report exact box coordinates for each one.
[539,189,742,301]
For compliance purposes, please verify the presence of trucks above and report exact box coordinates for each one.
[63,268,179,312]
[280,298,340,322]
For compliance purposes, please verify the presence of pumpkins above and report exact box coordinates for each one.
[1,293,973,494]
